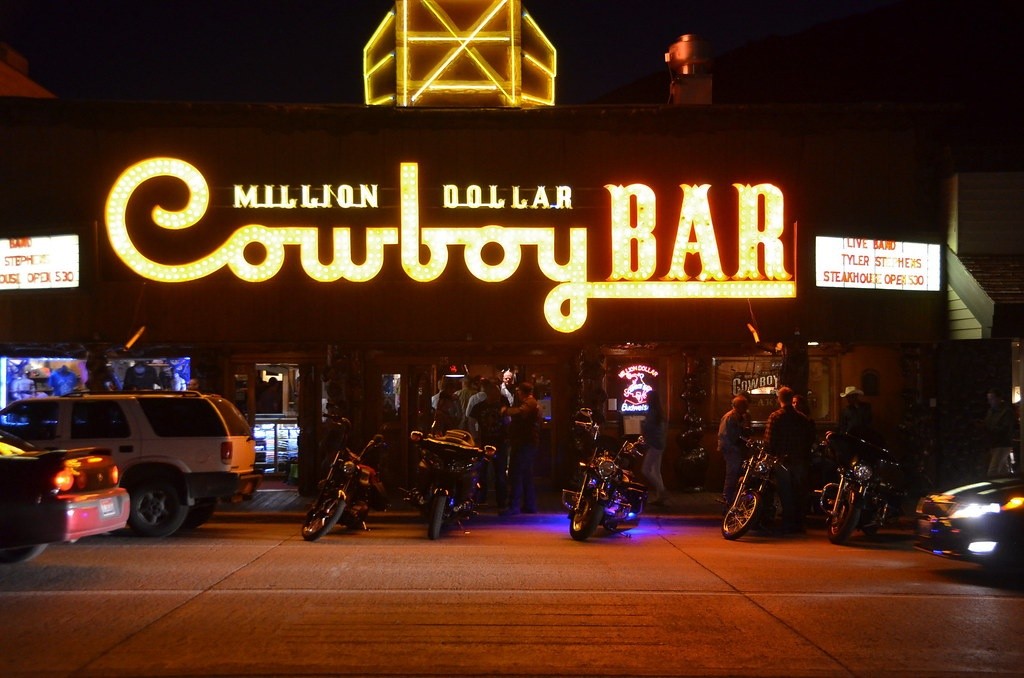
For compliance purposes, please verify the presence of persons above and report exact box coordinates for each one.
[13,370,34,400]
[837,386,872,432]
[122,360,157,391]
[49,365,77,396]
[716,390,752,516]
[431,371,543,515]
[976,388,1016,477]
[640,391,665,505]
[763,387,815,533]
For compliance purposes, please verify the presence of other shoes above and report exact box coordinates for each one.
[647,499,664,506]
[497,506,518,515]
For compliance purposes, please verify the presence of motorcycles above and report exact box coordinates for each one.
[300,403,393,542]
[813,428,900,546]
[408,428,498,541]
[561,407,649,542]
[716,433,790,540]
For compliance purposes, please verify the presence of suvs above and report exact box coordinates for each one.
[0,388,265,538]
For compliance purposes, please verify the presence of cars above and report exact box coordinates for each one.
[0,429,133,565]
[912,476,1023,570]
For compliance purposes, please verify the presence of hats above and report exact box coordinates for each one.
[840,386,863,400]
